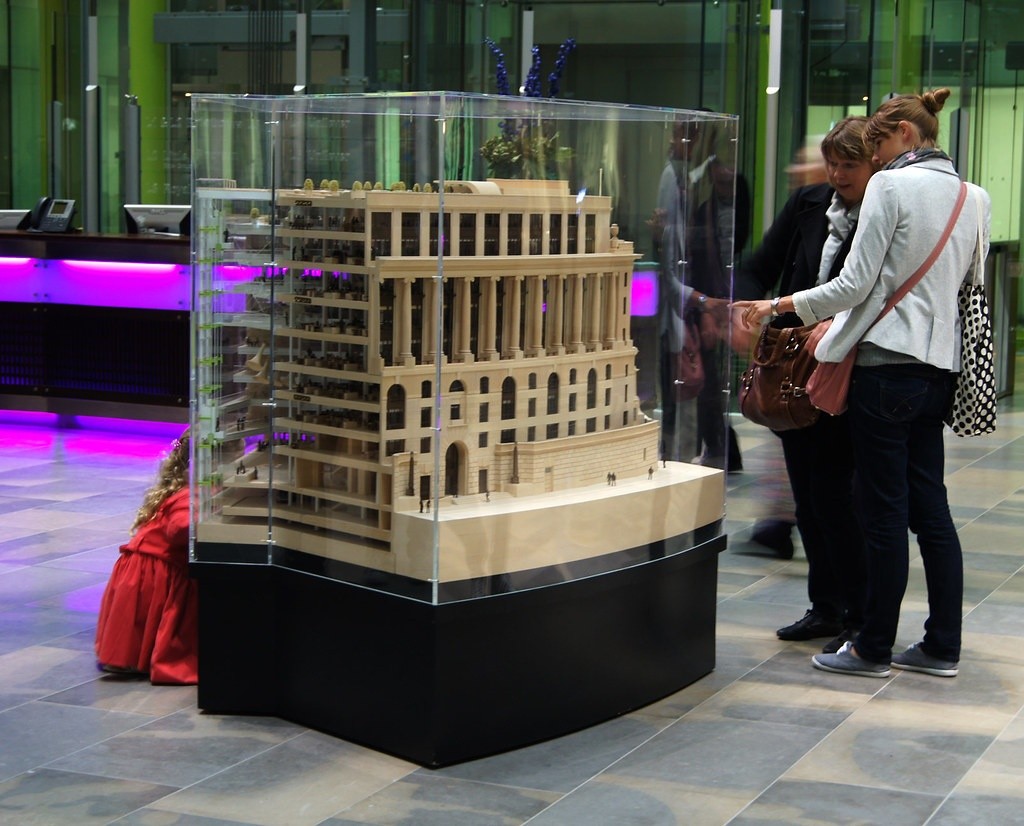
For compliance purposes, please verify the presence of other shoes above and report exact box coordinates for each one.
[691,455,742,471]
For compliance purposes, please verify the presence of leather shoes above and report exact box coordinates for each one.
[822,632,856,653]
[776,609,843,641]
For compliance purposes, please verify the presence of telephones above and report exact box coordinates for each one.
[26,196,77,233]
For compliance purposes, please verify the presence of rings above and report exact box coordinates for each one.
[746,308,749,313]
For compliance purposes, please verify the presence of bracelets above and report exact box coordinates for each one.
[699,294,707,306]
[772,297,780,316]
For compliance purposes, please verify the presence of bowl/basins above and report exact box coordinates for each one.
[124,204,192,225]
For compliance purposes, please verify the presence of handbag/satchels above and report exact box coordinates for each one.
[955,183,998,438]
[674,324,704,400]
[804,347,856,416]
[740,316,834,431]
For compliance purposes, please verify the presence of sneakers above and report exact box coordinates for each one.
[891,640,959,676]
[812,642,892,678]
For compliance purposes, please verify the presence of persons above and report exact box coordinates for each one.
[95,425,246,684]
[731,90,989,680]
[645,109,751,471]
